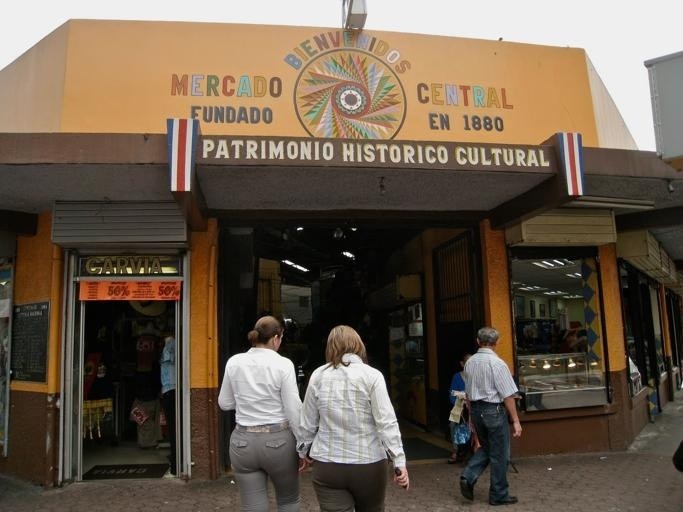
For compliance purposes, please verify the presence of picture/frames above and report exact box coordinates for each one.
[530,300,535,317]
[539,304,545,317]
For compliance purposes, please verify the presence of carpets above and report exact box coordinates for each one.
[299,437,454,467]
[82,464,169,480]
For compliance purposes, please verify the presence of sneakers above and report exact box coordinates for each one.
[460,479,473,500]
[497,496,518,504]
[448,453,464,463]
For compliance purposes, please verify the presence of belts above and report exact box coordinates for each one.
[236,423,286,433]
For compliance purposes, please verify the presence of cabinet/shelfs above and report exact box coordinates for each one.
[517,352,609,413]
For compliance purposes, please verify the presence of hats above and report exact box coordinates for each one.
[478,327,499,343]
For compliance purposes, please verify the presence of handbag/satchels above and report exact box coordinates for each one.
[450,416,471,445]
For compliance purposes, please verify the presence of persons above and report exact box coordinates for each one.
[448,354,480,462]
[217,315,306,512]
[296,326,409,512]
[459,326,522,505]
[160,336,182,478]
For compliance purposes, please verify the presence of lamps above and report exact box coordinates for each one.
[528,356,598,369]
[342,0,367,31]
